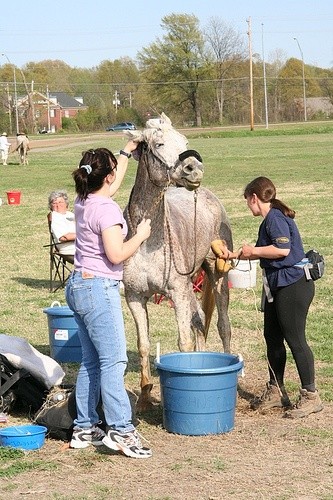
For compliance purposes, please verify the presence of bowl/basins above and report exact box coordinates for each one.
[0,425,47,451]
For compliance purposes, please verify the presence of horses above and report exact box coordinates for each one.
[121,111,234,413]
[16,135,31,165]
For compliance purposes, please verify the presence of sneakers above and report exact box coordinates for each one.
[101,429,153,458]
[285,386,324,418]
[70,420,106,448]
[253,382,291,410]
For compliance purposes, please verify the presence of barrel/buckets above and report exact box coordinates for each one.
[153,352,244,436]
[43,301,82,364]
[228,258,259,289]
[6,192,21,204]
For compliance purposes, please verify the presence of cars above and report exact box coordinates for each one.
[106,122,135,131]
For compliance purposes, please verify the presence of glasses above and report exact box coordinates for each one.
[82,149,96,155]
[50,202,64,207]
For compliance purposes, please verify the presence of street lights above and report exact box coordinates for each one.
[1,53,19,134]
[294,38,307,120]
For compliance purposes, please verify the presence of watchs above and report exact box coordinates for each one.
[120,150,131,159]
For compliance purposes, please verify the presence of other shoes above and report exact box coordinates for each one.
[3,163,7,165]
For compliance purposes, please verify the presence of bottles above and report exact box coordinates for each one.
[51,392,64,403]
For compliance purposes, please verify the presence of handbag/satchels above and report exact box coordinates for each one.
[293,249,325,281]
[37,387,107,442]
[0,354,49,419]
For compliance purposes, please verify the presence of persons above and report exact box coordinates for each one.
[0,132,11,165]
[66,140,152,458]
[48,192,77,264]
[219,176,323,418]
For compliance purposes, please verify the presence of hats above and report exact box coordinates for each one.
[1,132,7,135]
